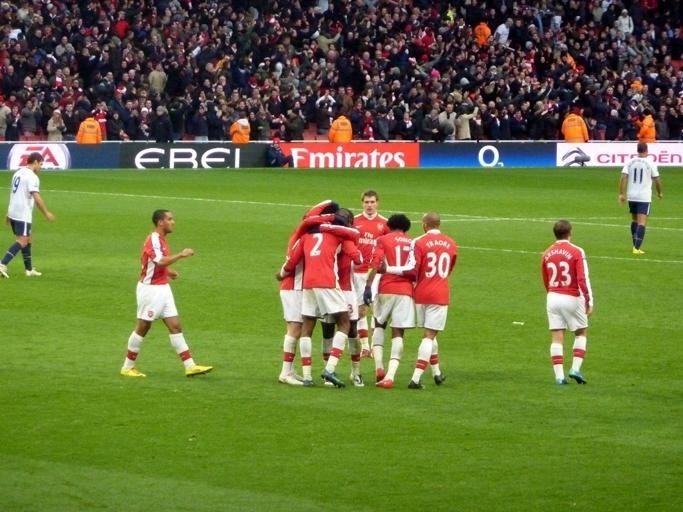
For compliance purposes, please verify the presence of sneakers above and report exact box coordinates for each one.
[0,261,9,278]
[557,377,567,384]
[25,269,42,276]
[568,368,587,384]
[185,365,213,376]
[121,367,146,377]
[633,247,645,254]
[278,347,446,389]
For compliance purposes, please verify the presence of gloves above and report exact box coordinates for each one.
[364,286,372,306]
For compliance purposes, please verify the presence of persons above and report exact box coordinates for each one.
[274,187,364,389]
[540,219,593,385]
[364,214,417,387]
[617,142,663,255]
[119,209,213,378]
[375,211,458,390]
[1,153,56,278]
[350,192,392,359]
[0,0,683,143]
